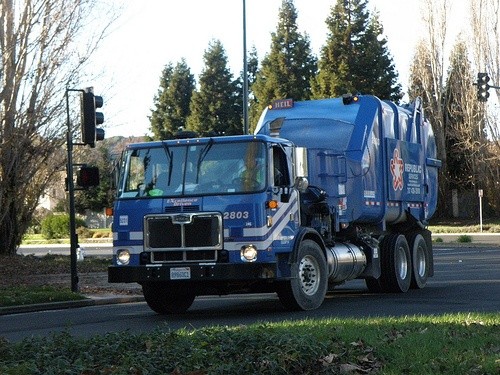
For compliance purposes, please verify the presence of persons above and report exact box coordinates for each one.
[237,150,282,192]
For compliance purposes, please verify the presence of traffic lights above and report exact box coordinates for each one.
[77,167,99,187]
[84,91,104,148]
[476,73,489,102]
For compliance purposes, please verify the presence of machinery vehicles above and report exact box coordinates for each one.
[104,89,442,310]
